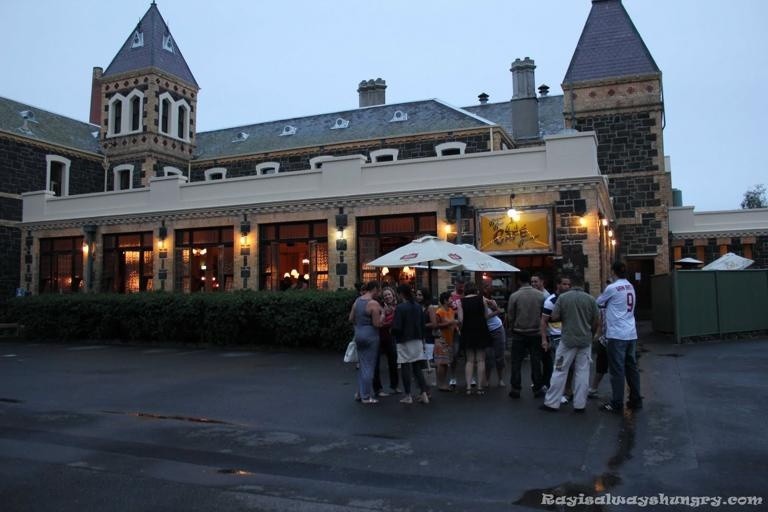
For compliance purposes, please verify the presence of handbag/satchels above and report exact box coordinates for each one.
[344,340,359,364]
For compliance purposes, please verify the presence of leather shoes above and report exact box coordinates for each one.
[509,391,521,399]
[534,391,549,398]
[574,408,585,414]
[539,405,559,412]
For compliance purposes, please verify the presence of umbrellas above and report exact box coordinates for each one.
[364,234,521,303]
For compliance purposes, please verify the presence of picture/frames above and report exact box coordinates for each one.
[474,204,555,256]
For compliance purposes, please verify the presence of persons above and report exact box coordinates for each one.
[280,276,292,290]
[345,260,643,416]
[290,274,308,289]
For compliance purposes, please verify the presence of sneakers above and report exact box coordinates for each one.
[600,404,623,414]
[626,401,643,409]
[354,379,489,406]
[586,389,600,400]
[498,382,506,388]
[560,396,569,404]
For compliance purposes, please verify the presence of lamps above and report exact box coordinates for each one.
[506,193,521,222]
